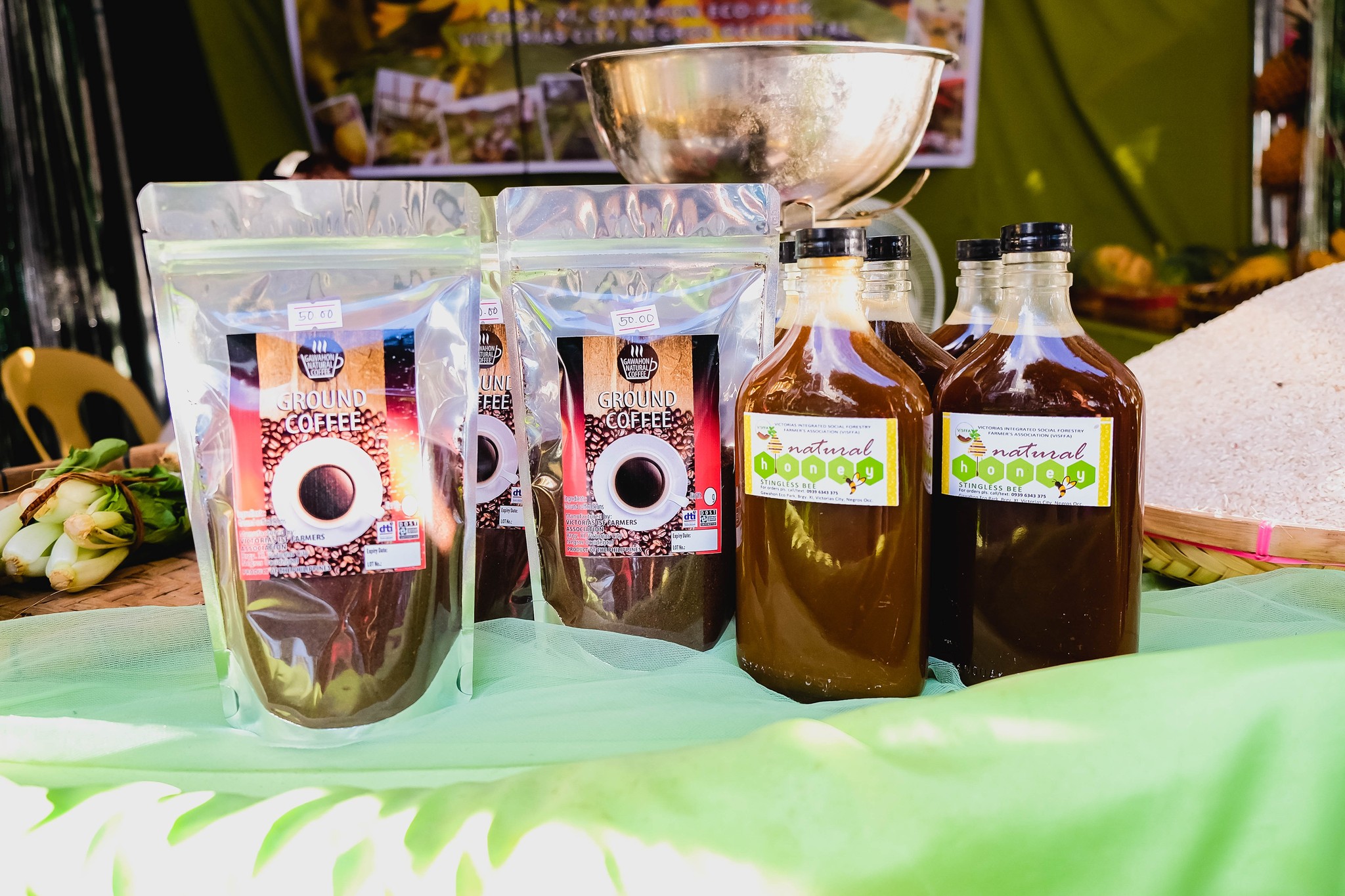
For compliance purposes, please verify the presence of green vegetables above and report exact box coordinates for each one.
[0,437,199,595]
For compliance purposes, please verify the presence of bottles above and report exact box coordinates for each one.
[863,235,958,395]
[735,225,931,707]
[929,239,1001,359]
[935,222,1147,689]
[773,238,801,350]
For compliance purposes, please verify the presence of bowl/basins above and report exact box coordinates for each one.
[565,39,960,226]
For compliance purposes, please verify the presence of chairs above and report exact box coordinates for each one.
[1,347,162,462]
